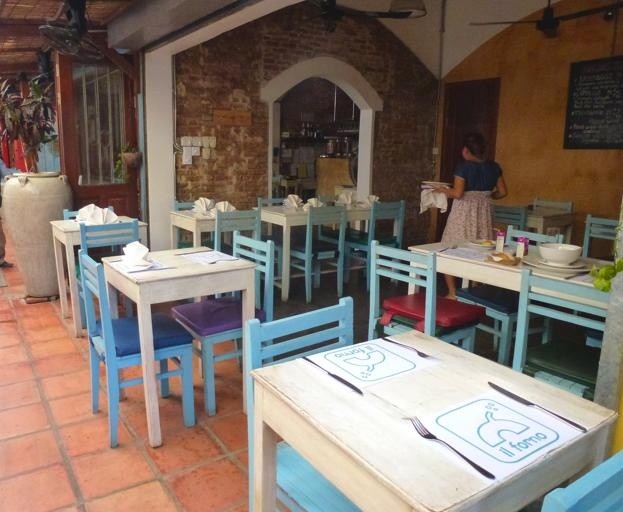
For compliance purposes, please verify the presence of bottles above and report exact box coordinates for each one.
[302,119,320,138]
[495,229,505,253]
[516,236,530,258]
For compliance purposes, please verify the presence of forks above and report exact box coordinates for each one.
[381,336,441,364]
[439,245,459,252]
[408,418,496,480]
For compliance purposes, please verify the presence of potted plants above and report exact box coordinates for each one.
[113,142,143,183]
[0,70,73,297]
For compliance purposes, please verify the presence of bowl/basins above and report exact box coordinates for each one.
[539,243,583,265]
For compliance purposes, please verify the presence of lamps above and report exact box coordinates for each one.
[389,0,428,18]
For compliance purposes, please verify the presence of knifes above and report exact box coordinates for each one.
[488,380,589,434]
[565,272,590,279]
[303,355,363,396]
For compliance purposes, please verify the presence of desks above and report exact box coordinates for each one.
[279,179,299,198]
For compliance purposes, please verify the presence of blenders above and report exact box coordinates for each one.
[320,136,341,157]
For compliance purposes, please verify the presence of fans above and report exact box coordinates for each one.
[468,0,623,40]
[283,0,413,32]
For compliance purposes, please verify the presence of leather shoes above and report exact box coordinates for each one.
[0,261,12,267]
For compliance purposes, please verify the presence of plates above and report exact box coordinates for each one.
[422,180,451,186]
[538,260,584,268]
[523,260,590,274]
[468,237,508,248]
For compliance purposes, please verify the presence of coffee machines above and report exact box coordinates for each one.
[342,136,354,158]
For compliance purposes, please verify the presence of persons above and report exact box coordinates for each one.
[0,156,14,269]
[431,132,508,300]
